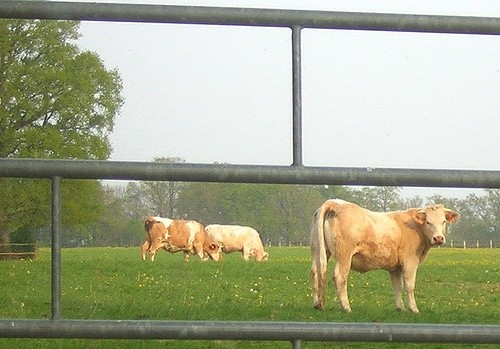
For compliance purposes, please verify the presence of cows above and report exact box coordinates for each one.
[204,223,271,263]
[141,216,225,264]
[309,197,460,315]
